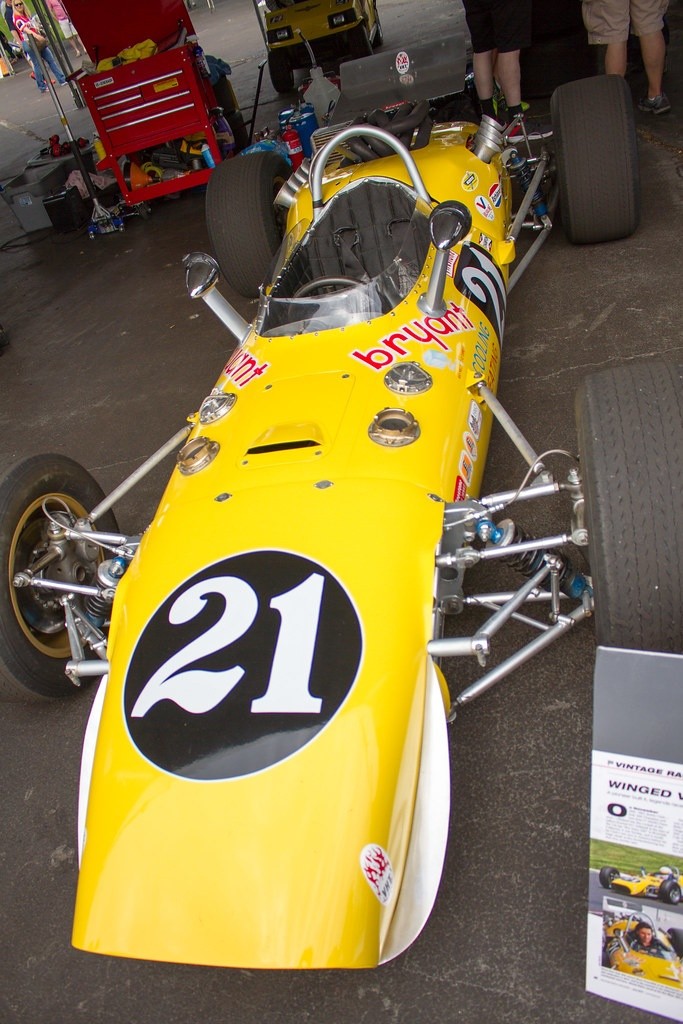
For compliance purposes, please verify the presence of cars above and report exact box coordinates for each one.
[260,0,386,96]
[604,911,683,990]
[599,860,683,906]
[0,29,683,973]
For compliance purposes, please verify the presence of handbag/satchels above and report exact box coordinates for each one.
[27,38,49,52]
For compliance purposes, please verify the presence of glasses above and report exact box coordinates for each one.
[15,3,23,6]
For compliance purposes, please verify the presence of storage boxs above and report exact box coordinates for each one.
[27,143,97,175]
[0,163,63,232]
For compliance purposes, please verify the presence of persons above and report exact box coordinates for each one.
[462,0,531,122]
[0,0,88,95]
[630,922,667,962]
[582,0,672,116]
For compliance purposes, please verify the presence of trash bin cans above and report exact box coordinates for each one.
[28,145,95,175]
[0,162,75,234]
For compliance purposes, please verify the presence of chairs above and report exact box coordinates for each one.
[298,124,430,302]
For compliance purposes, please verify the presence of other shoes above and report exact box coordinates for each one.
[84,50,87,54]
[61,81,67,86]
[40,87,50,91]
[44,79,56,83]
[31,71,36,80]
[76,52,82,57]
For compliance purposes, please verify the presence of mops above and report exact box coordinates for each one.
[28,33,125,239]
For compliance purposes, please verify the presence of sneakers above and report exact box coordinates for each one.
[504,122,552,143]
[637,91,671,114]
[482,112,507,131]
